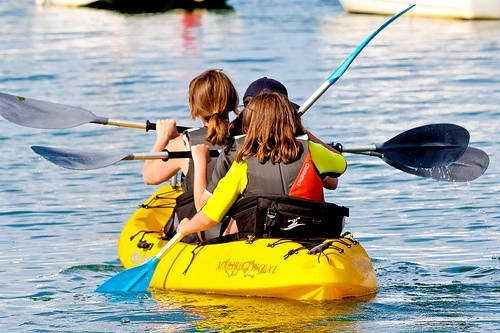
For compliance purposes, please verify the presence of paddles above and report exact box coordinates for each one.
[94,4,416,293]
[31,122,470,168]
[0,91,489,182]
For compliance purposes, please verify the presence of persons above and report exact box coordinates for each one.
[190,75,338,244]
[177,89,346,245]
[142,68,240,245]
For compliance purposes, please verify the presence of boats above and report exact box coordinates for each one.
[117,172,380,303]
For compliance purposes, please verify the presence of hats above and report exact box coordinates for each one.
[243,76,300,108]
